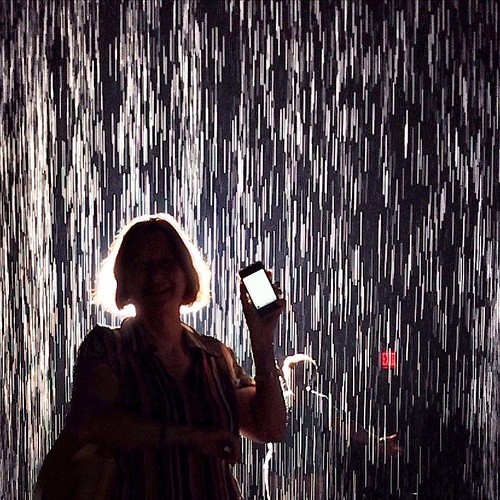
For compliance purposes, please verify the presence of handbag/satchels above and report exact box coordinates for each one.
[33,326,122,500]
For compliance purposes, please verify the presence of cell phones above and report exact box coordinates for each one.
[239,261,281,316]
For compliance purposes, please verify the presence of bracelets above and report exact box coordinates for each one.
[252,362,284,386]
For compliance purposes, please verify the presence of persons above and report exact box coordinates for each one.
[261,354,406,499]
[36,215,286,499]
